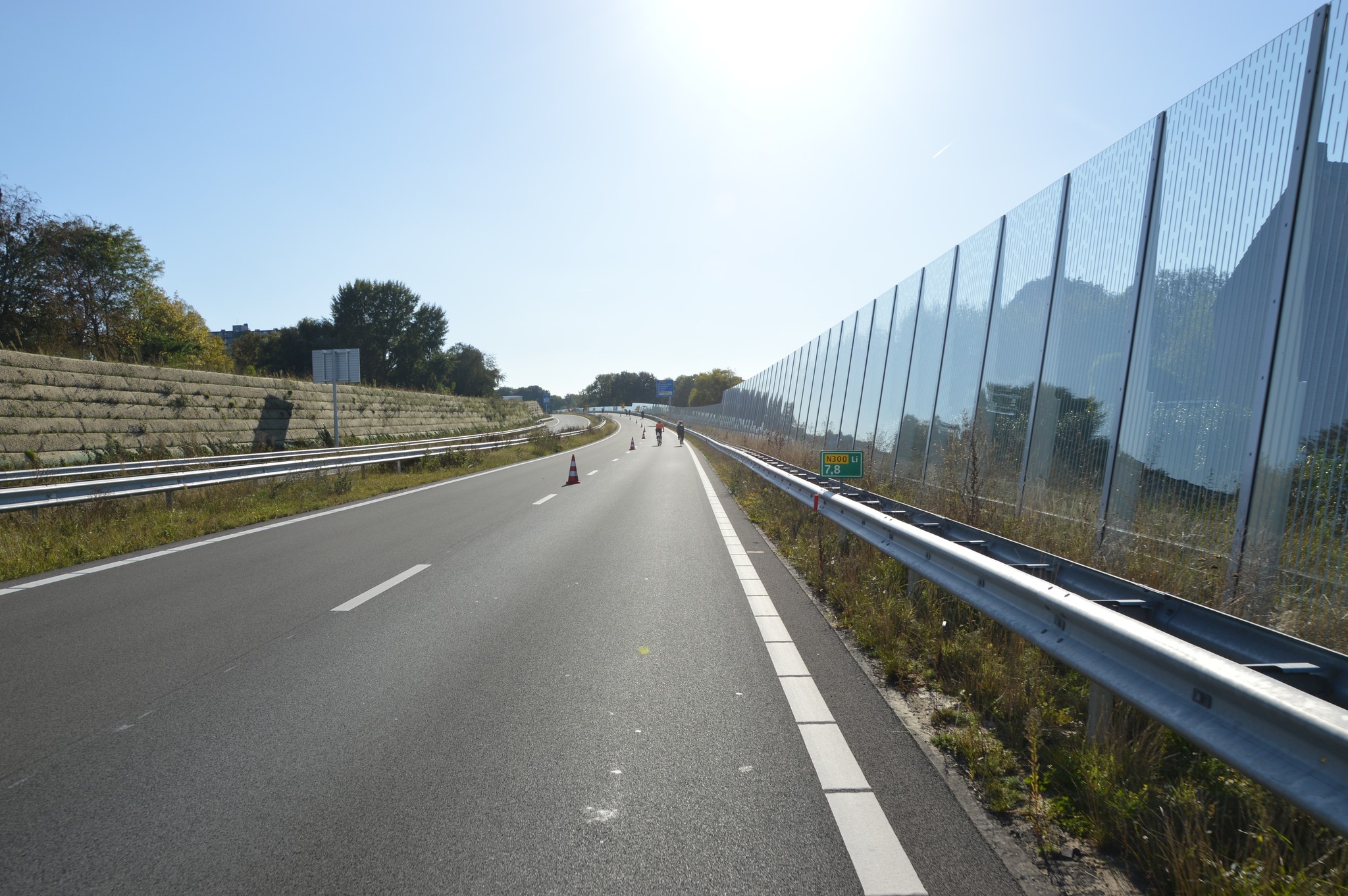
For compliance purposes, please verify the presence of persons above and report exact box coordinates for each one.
[676,421,684,445]
[655,421,664,444]
[628,409,631,415]
[601,407,605,413]
[582,409,584,412]
[641,410,644,418]
[625,410,628,415]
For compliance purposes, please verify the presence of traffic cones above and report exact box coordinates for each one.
[607,412,608,415]
[629,437,636,450]
[641,431,646,439]
[641,423,643,428]
[620,414,621,417]
[643,426,646,432]
[636,419,637,423]
[629,417,631,420]
[566,454,580,484]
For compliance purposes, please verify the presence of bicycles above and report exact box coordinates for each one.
[657,431,664,446]
[641,413,644,419]
[677,431,685,447]
[626,411,630,416]
